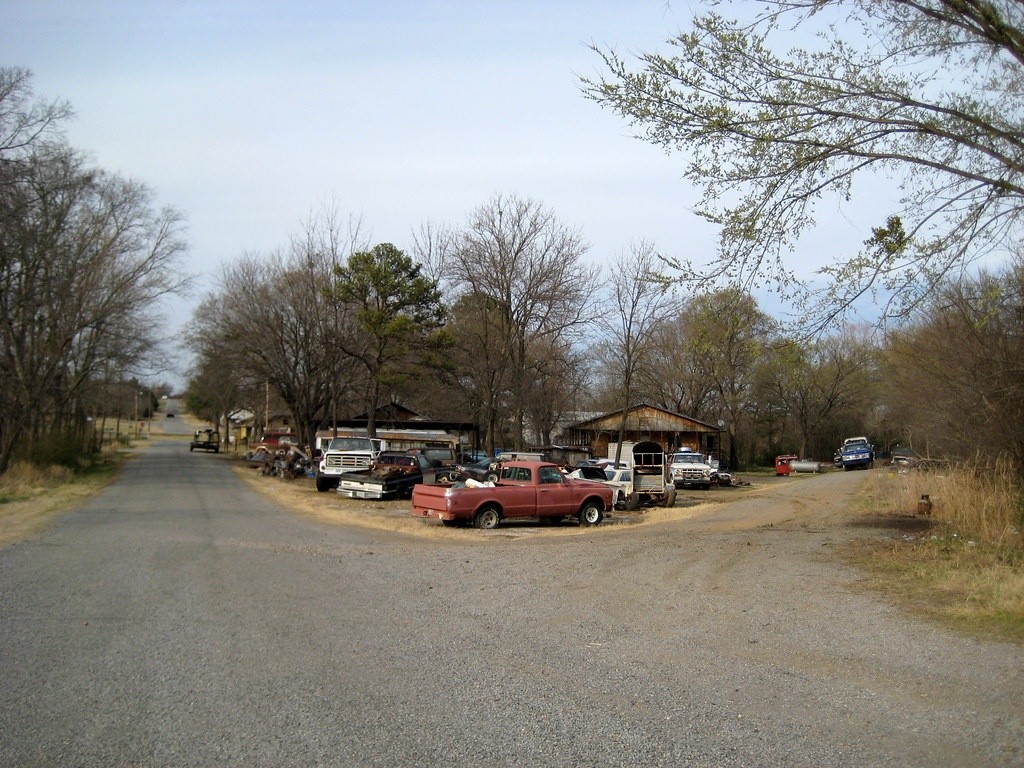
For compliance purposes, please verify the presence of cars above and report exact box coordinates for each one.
[278,437,300,450]
[559,459,634,505]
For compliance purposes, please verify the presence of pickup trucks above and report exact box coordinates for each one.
[317,437,378,492]
[842,437,872,471]
[413,461,613,528]
[337,452,455,502]
[404,447,547,483]
[666,447,711,489]
